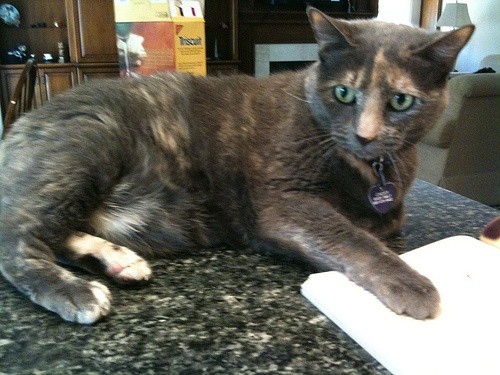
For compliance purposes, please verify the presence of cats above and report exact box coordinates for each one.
[0,5,476,327]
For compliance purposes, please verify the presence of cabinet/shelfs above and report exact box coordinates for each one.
[0,0,121,121]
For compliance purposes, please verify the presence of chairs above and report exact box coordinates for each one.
[0,58,38,137]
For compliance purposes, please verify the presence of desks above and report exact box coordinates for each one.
[0,176,500,374]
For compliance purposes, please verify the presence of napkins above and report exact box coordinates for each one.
[302,234,500,374]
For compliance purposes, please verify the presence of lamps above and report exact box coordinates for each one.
[435,2,474,74]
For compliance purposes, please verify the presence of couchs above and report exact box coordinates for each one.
[418,72,500,206]
